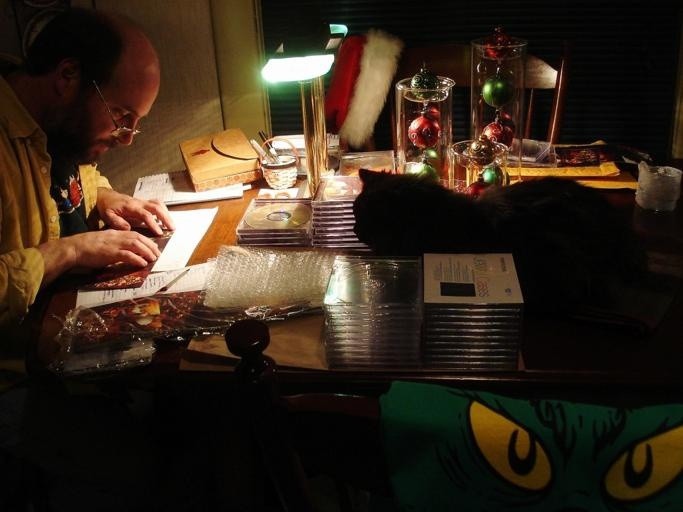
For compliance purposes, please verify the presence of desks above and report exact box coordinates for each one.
[36,150,682,391]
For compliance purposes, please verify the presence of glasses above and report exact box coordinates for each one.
[93,80,144,138]
[224,318,277,389]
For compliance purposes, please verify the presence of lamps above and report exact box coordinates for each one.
[261,31,343,189]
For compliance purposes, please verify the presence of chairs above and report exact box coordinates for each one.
[391,45,567,151]
[272,394,384,511]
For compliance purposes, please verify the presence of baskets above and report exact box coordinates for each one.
[635,161,683,211]
[259,138,300,189]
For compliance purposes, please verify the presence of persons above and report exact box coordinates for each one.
[0,1,176,319]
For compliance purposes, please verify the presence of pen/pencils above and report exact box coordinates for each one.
[160,269,190,291]
[249,131,282,163]
